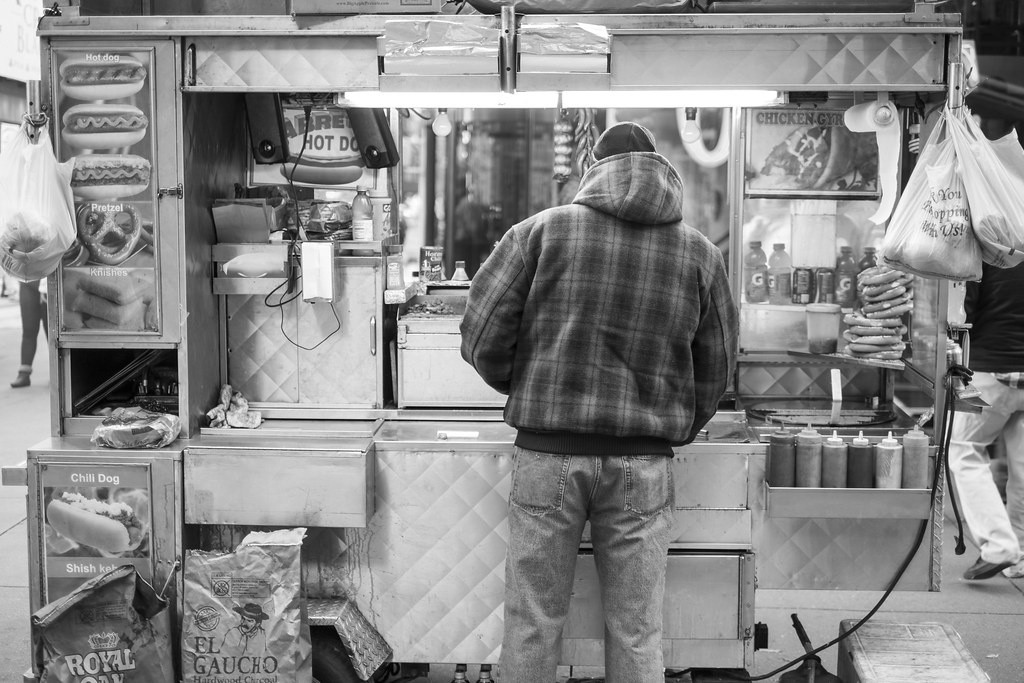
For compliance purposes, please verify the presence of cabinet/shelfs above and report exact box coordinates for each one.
[218,265,377,409]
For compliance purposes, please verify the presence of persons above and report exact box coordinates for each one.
[11,275,48,387]
[459,122,739,683]
[455,189,485,281]
[946,264,1024,579]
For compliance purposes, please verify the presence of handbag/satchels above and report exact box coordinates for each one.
[0,116,80,281]
[945,100,1024,269]
[882,100,982,281]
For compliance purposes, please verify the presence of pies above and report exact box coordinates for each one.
[754,123,878,191]
[842,265,914,361]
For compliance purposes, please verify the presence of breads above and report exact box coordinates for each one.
[62,200,159,334]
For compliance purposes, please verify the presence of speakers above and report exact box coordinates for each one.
[347,106,399,168]
[245,91,290,165]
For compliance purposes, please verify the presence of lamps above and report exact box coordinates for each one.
[332,90,789,109]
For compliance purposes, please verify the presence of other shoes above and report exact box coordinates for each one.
[10,365,31,387]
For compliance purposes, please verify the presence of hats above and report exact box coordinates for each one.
[592,122,656,161]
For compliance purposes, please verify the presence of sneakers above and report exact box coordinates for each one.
[965,554,1015,579]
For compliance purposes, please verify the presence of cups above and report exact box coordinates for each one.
[806,303,841,354]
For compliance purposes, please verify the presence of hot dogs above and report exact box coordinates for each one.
[58,54,151,199]
[279,152,368,185]
[47,490,148,553]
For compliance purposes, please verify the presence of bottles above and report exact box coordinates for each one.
[450,261,469,282]
[847,430,874,488]
[386,244,405,290]
[769,421,796,489]
[876,430,903,487]
[858,246,878,273]
[368,188,391,253]
[768,243,792,305]
[796,423,846,488]
[835,246,858,307]
[352,185,374,256]
[903,424,929,489]
[744,241,767,302]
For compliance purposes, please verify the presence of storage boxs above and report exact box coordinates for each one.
[839,618,991,683]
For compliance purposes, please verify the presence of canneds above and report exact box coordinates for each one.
[792,267,834,305]
